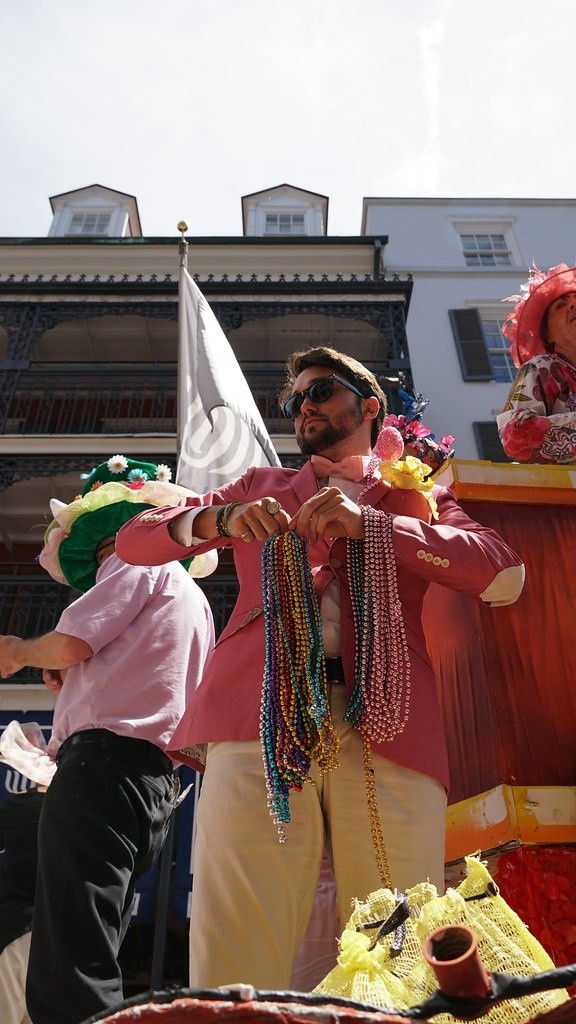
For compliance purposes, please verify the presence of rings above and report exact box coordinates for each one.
[267,501,282,514]
[241,531,251,538]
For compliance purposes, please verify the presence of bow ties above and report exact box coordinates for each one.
[311,455,363,482]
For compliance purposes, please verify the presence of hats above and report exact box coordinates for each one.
[500,258,576,368]
[36,454,218,594]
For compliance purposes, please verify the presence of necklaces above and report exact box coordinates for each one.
[342,505,411,889]
[315,458,382,504]
[261,531,338,843]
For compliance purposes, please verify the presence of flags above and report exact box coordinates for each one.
[175,268,281,494]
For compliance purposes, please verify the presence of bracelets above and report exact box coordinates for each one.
[216,502,241,537]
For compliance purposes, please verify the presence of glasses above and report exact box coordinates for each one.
[283,374,366,422]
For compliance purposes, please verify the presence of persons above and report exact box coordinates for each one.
[116,348,526,997]
[498,264,576,466]
[0,930,33,1024]
[0,455,215,1024]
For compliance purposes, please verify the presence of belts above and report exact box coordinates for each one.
[55,733,174,779]
[315,656,344,683]
